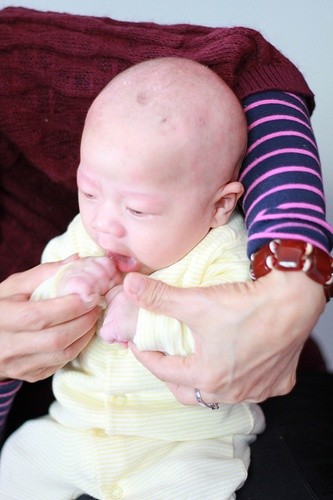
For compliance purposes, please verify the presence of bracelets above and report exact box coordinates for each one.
[249,240,333,305]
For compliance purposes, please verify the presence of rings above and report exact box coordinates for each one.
[194,388,219,412]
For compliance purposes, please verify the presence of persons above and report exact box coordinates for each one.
[1,0,333,500]
[0,58,268,500]
